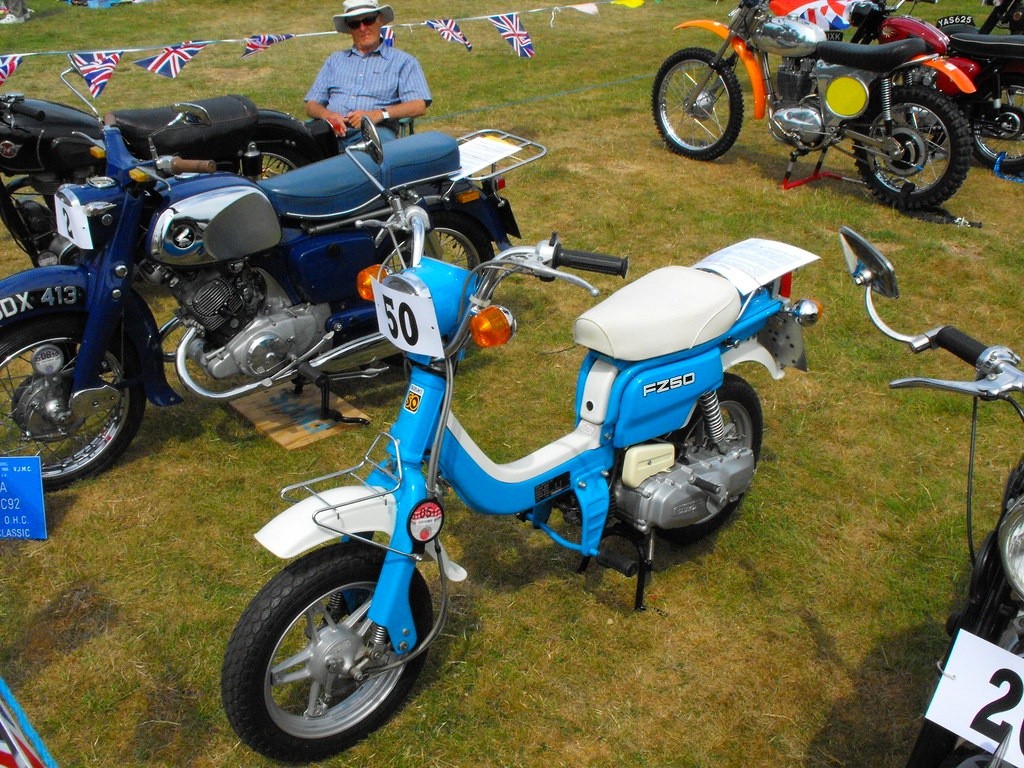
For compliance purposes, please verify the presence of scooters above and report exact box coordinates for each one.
[837,224,1024,768]
[219,116,824,765]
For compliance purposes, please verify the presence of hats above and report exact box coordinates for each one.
[332,0,395,33]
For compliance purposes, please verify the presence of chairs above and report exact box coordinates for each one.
[306,116,416,139]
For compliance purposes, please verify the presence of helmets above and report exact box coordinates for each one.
[847,1,880,30]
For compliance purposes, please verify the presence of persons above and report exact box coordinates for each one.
[306,0,428,154]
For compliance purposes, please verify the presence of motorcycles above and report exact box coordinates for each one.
[649,0,979,213]
[0,100,548,496]
[846,0,1024,177]
[0,91,341,268]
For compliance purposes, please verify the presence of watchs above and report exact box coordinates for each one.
[380,107,389,125]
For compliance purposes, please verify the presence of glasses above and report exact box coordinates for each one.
[344,14,383,31]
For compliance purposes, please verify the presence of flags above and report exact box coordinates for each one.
[132,40,208,79]
[766,0,861,31]
[424,19,472,52]
[0,56,23,86]
[379,26,397,47]
[74,51,124,98]
[0,696,46,768]
[487,14,535,58]
[240,34,296,60]
